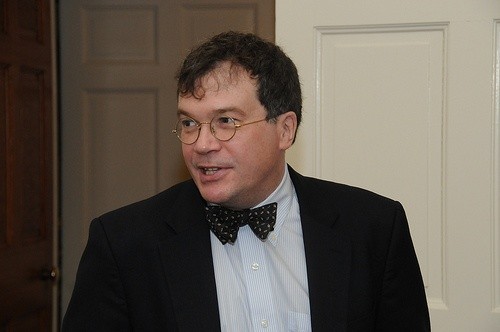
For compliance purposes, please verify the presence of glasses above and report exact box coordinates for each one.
[171,114,278,145]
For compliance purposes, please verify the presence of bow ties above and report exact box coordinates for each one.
[205,202,278,245]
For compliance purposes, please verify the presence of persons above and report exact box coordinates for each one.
[59,30,433,332]
